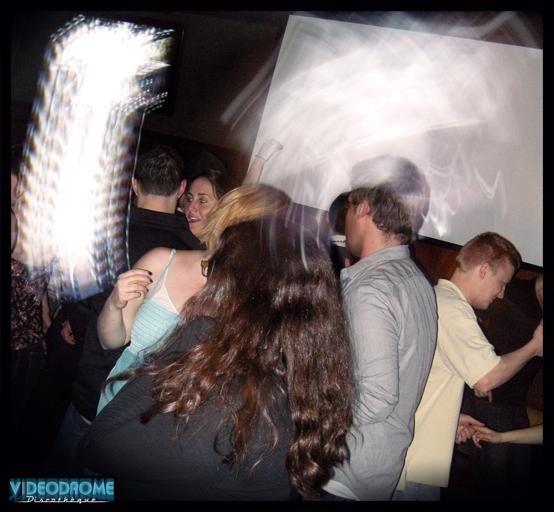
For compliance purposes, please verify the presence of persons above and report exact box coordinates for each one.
[5,142,544,508]
